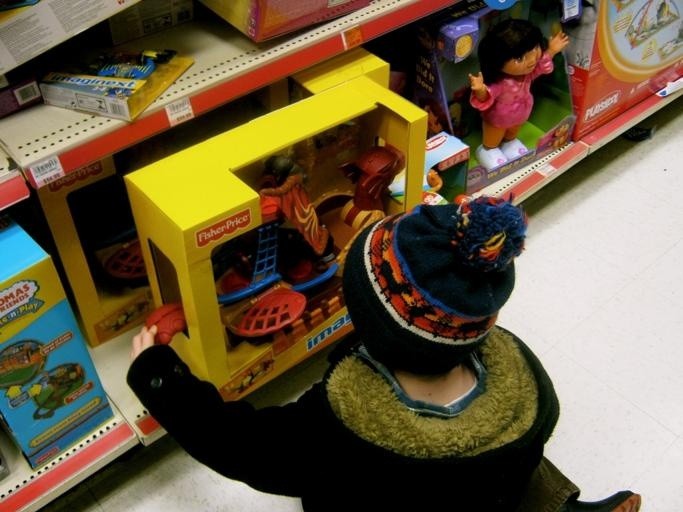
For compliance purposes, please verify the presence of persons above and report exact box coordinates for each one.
[124,192,643,511]
[412,0,578,192]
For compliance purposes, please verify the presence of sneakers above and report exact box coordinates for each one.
[565,491,641,512]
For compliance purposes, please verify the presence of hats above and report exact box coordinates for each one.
[342,195,526,374]
